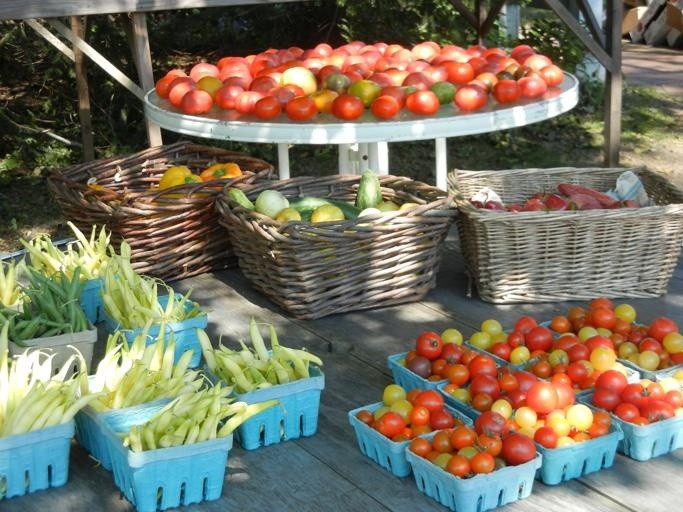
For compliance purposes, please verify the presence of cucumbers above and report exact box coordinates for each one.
[226,169,383,221]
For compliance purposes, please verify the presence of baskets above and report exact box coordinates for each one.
[212,172,459,320]
[43,137,275,285]
[446,166,683,305]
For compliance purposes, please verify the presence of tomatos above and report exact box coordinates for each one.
[156,41,564,121]
[356,297,683,476]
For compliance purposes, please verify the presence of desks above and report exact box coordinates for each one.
[143,66,582,195]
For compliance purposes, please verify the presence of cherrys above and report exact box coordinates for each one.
[470,184,639,213]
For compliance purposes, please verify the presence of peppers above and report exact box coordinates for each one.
[79,161,252,220]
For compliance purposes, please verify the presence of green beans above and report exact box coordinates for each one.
[0,221,326,512]
